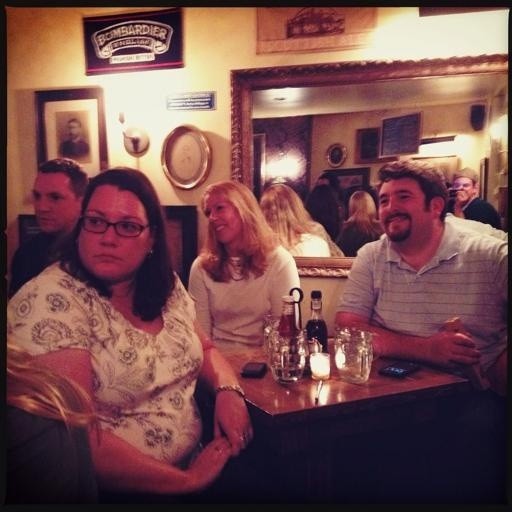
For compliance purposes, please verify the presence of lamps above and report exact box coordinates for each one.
[117,109,150,155]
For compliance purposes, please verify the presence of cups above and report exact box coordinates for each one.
[334,328,384,386]
[269,328,322,384]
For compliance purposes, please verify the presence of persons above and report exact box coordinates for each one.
[7,165,255,512]
[448,168,501,231]
[317,173,339,189]
[5,325,103,512]
[8,157,89,302]
[335,189,386,258]
[188,179,301,360]
[259,183,345,257]
[57,119,89,158]
[333,157,508,400]
[305,184,340,242]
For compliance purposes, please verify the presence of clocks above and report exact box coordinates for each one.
[325,143,347,168]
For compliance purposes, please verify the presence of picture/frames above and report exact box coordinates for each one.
[33,85,108,180]
[160,205,199,291]
[479,158,487,204]
[353,126,385,164]
[160,124,212,192]
[322,167,369,194]
[377,111,423,158]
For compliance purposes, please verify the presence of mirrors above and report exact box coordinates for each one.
[226,52,508,278]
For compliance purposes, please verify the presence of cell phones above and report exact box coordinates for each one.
[240,361,267,379]
[378,360,421,378]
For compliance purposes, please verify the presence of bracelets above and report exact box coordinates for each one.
[215,384,245,396]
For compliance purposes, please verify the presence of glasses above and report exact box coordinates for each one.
[79,216,149,238]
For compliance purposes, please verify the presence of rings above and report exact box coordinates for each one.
[239,435,246,440]
[215,447,222,454]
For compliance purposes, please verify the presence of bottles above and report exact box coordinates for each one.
[277,290,328,352]
